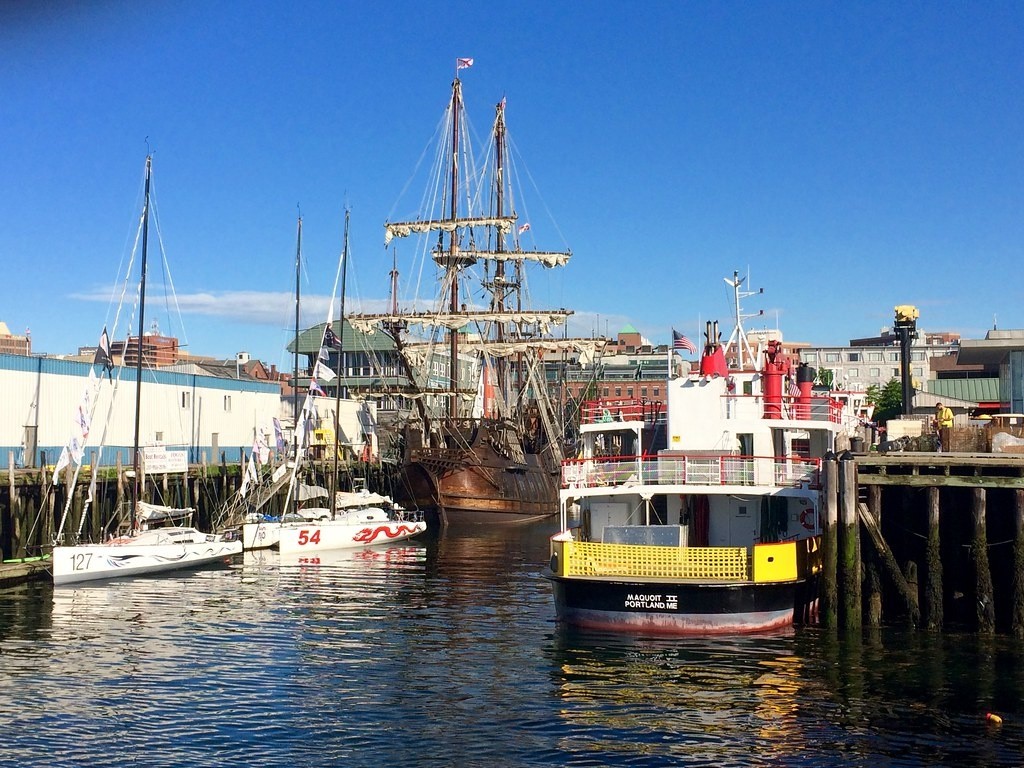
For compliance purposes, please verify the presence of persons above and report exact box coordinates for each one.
[933,402,954,451]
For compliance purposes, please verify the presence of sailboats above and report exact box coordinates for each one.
[0,71,880,637]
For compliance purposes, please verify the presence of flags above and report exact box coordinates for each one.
[50,325,114,487]
[242,410,286,497]
[789,379,801,396]
[289,302,341,458]
[672,328,698,355]
